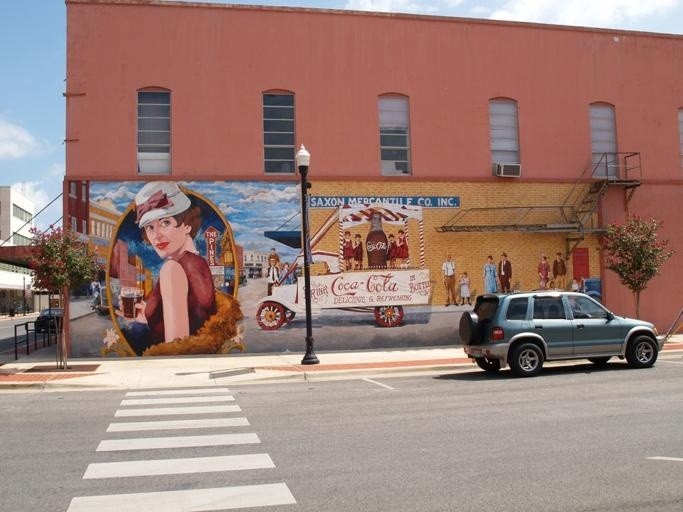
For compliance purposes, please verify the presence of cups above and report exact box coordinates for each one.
[120,286,145,320]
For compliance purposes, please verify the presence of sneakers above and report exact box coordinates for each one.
[446,302,472,307]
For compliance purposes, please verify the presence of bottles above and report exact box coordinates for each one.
[366,212,389,269]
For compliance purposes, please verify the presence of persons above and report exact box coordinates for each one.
[536,256,551,289]
[110,180,218,348]
[265,258,280,296]
[482,255,498,293]
[393,228,409,269]
[276,261,293,285]
[570,277,579,292]
[440,255,459,307]
[496,252,512,294]
[384,233,398,268]
[551,252,566,291]
[342,231,354,271]
[266,247,280,265]
[458,272,472,306]
[350,234,363,271]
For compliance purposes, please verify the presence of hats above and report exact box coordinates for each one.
[135,181,192,229]
[501,252,508,256]
[344,231,351,236]
[355,234,361,239]
[388,234,395,238]
[398,230,404,234]
[269,259,276,263]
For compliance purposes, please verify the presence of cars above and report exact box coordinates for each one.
[458,291,658,378]
[34,308,62,333]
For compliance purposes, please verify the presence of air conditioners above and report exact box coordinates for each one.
[496,162,521,177]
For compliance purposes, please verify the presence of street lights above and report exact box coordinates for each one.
[295,143,319,365]
